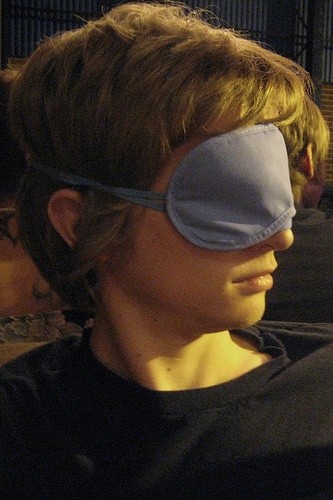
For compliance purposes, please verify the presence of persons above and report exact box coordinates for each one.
[1,2,333,500]
